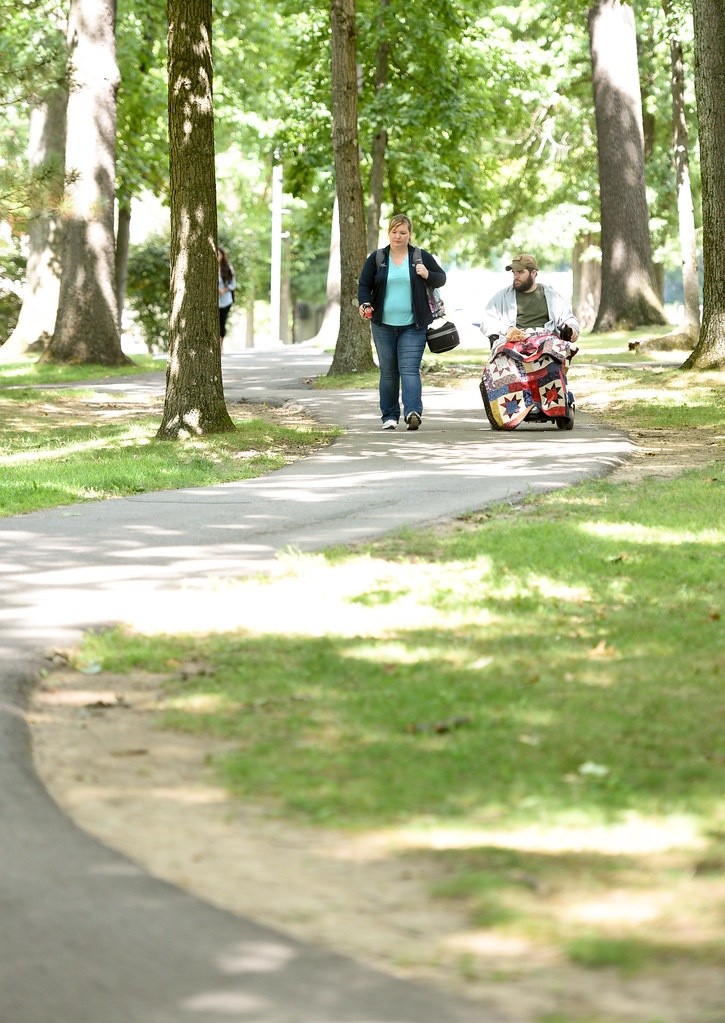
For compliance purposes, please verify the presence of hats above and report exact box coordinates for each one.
[506,254,537,271]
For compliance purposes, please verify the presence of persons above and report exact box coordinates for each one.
[219,247,237,347]
[481,252,580,345]
[358,214,447,431]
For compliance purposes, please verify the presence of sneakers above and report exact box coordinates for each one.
[382,419,398,430]
[406,410,421,430]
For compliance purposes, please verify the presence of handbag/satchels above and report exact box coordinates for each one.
[419,250,445,319]
[426,322,460,354]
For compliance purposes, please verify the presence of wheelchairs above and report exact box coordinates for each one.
[489,323,575,431]
